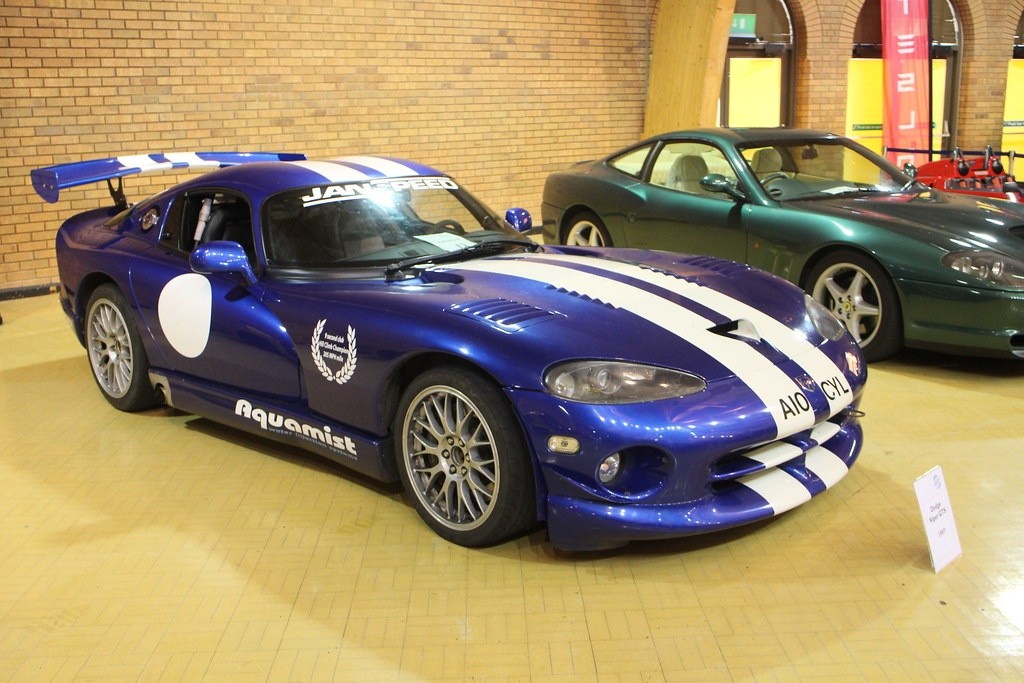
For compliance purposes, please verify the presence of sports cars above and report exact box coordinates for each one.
[32,149,868,555]
[541,128,1023,369]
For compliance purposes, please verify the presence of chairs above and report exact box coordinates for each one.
[666,155,727,199]
[199,205,299,266]
[751,148,783,188]
